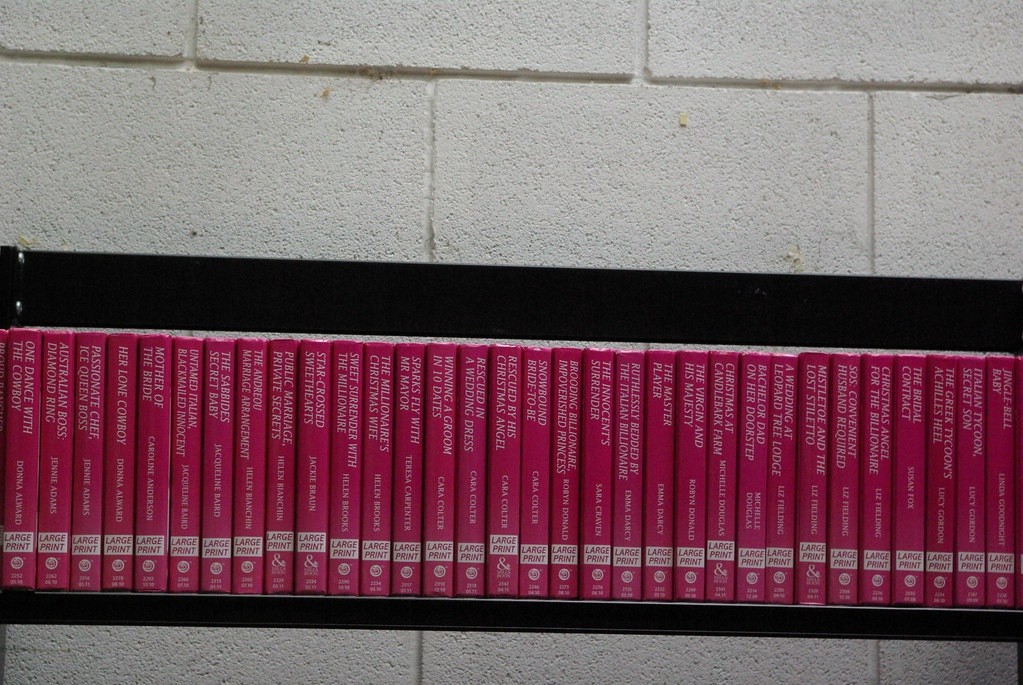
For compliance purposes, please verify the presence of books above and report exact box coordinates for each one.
[0,327,1023,609]
